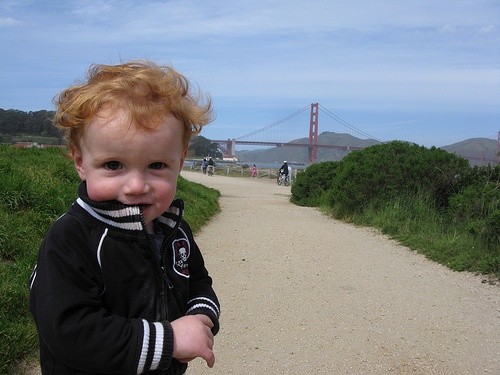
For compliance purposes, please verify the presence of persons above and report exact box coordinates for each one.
[279,161,288,181]
[251,164,257,177]
[202,158,207,174]
[207,156,215,175]
[27,58,221,375]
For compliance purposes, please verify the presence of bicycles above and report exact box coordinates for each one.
[277,169,289,186]
[207,167,214,177]
